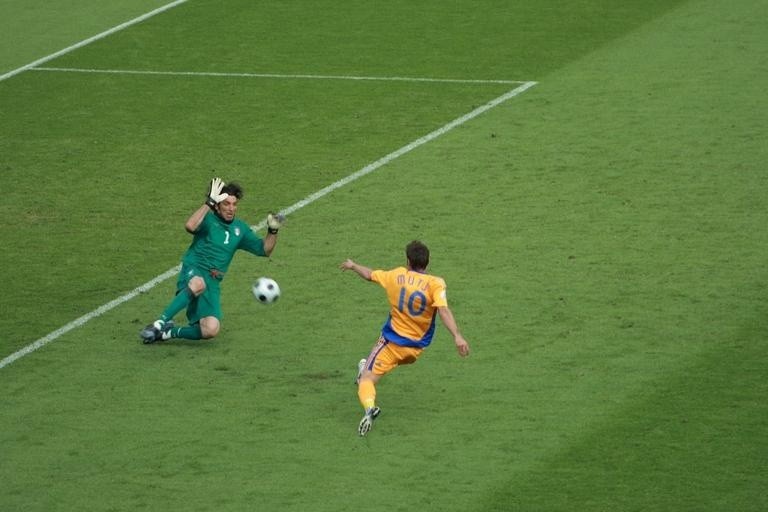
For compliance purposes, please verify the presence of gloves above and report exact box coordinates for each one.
[267,212,284,234]
[205,177,229,210]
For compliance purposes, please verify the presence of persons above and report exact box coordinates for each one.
[140,174,283,348]
[338,239,471,437]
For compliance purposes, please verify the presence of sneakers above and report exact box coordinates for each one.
[356,358,367,384]
[358,407,381,437]
[140,319,165,339]
[143,320,176,344]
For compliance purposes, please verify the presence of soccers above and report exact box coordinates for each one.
[253,276,279,303]
